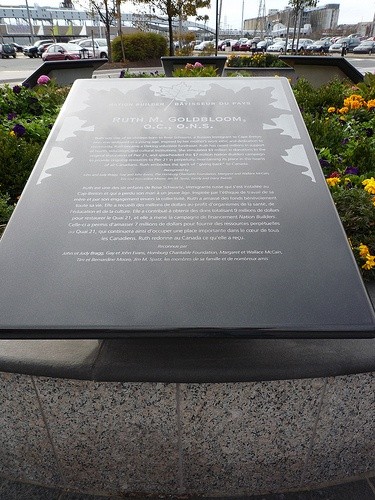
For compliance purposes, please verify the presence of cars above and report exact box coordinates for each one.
[12,42,23,52]
[69,38,108,58]
[232,37,375,53]
[37,42,92,61]
[174,38,234,50]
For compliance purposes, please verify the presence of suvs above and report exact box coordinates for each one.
[0,43,17,58]
[23,40,54,58]
